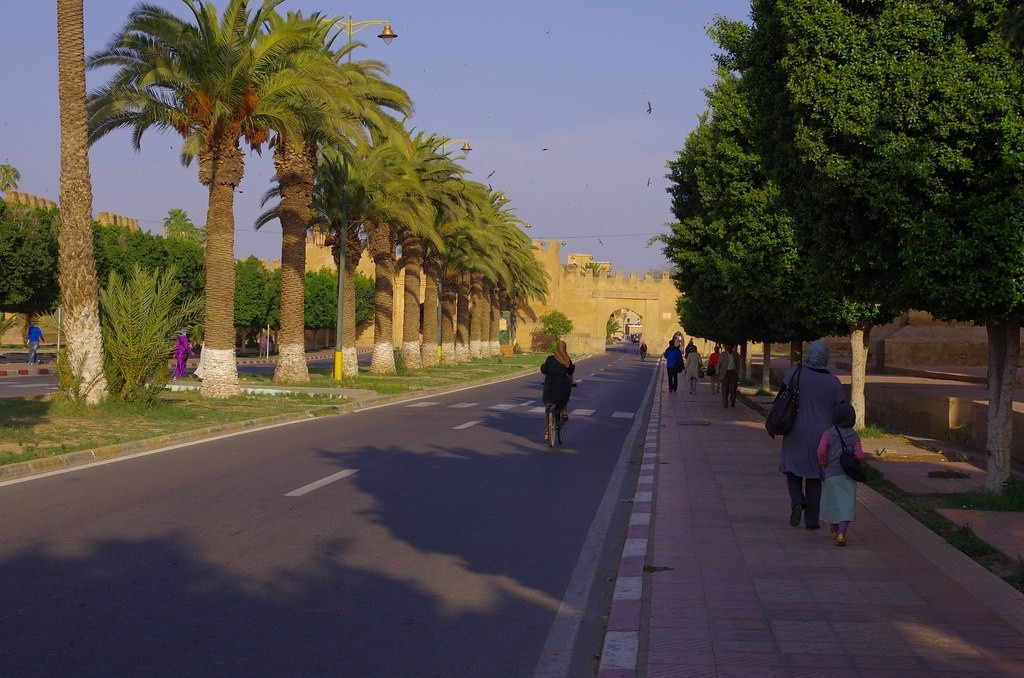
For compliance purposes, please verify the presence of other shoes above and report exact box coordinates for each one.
[669,389,673,392]
[543,430,550,440]
[173,374,177,380]
[730,402,735,408]
[37,358,42,363]
[722,404,728,408]
[561,414,569,423]
[673,388,677,392]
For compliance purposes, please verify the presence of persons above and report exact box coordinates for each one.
[633,334,636,344]
[817,401,864,547]
[173,328,194,380]
[716,342,739,408]
[665,340,683,392]
[260,335,267,358]
[769,340,844,531]
[685,342,702,394]
[27,320,45,365]
[675,338,680,347]
[640,342,647,362]
[708,347,720,394]
[540,340,576,440]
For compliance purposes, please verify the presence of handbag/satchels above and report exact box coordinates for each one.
[697,369,705,378]
[675,357,685,373]
[707,364,716,376]
[765,365,805,440]
[834,426,868,483]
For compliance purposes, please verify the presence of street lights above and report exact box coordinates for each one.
[321,11,397,381]
[436,136,473,363]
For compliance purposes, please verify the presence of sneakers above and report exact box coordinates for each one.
[789,504,803,528]
[805,522,821,531]
[689,390,693,395]
[831,532,846,546]
[693,391,697,395]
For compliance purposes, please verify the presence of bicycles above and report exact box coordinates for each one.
[541,381,576,447]
